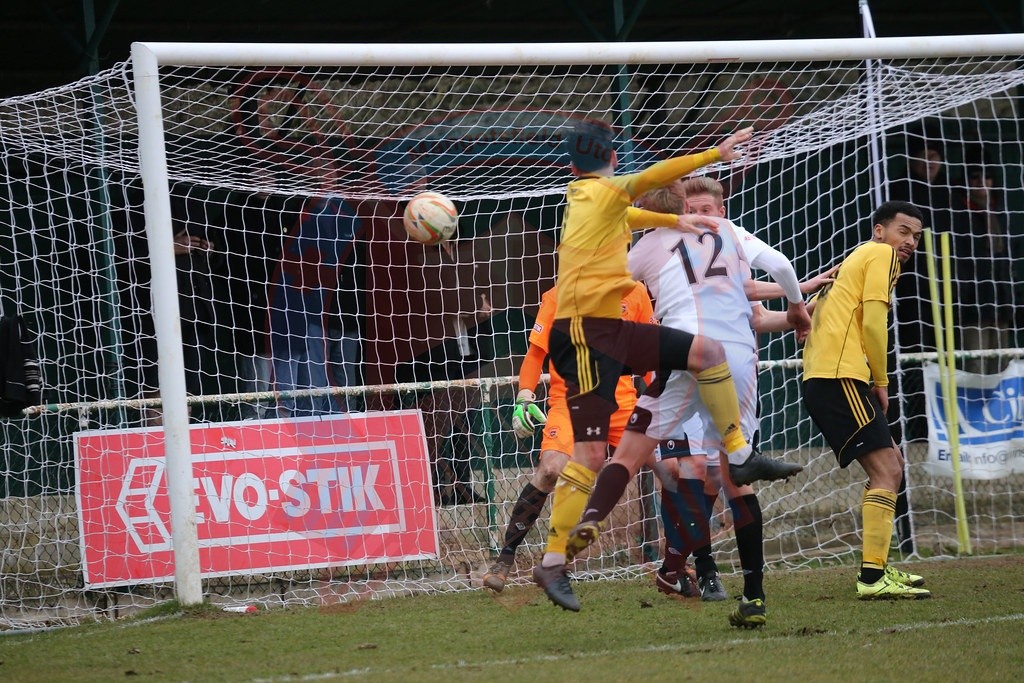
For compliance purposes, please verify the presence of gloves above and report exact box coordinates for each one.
[511,389,546,438]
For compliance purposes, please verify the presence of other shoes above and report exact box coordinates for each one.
[449,487,488,505]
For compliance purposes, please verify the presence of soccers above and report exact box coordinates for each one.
[403,191,459,245]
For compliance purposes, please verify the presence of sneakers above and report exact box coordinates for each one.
[857,564,926,587]
[656,569,701,599]
[534,561,581,612]
[564,521,607,563]
[728,449,803,488]
[855,574,931,600]
[695,565,727,602]
[684,562,698,578]
[729,594,766,629]
[483,560,512,592]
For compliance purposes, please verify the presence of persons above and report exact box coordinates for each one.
[482,118,814,630]
[800,201,932,600]
[891,145,1013,442]
[131,158,485,506]
